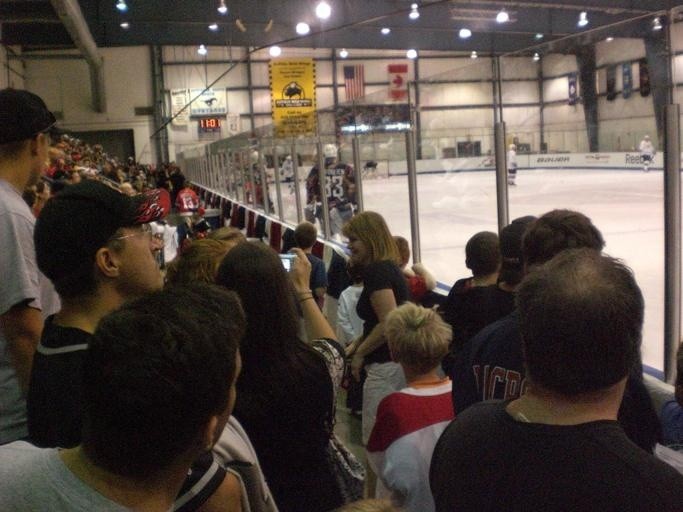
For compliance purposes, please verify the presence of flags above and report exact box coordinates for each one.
[388,63,408,101]
[568,58,651,105]
[344,64,365,103]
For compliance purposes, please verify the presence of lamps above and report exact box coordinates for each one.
[217,0,229,14]
[197,41,207,56]
[118,17,131,30]
[114,0,128,10]
[208,20,218,35]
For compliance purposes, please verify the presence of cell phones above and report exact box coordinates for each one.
[276,254,295,271]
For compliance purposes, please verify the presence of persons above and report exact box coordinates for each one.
[1,86,682,512]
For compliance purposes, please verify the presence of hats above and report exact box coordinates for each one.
[34,180,171,282]
[250,151,264,164]
[0,87,57,140]
[322,144,338,158]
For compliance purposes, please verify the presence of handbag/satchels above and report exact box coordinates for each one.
[327,431,368,501]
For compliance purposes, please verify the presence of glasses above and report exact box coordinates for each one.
[115,224,153,243]
